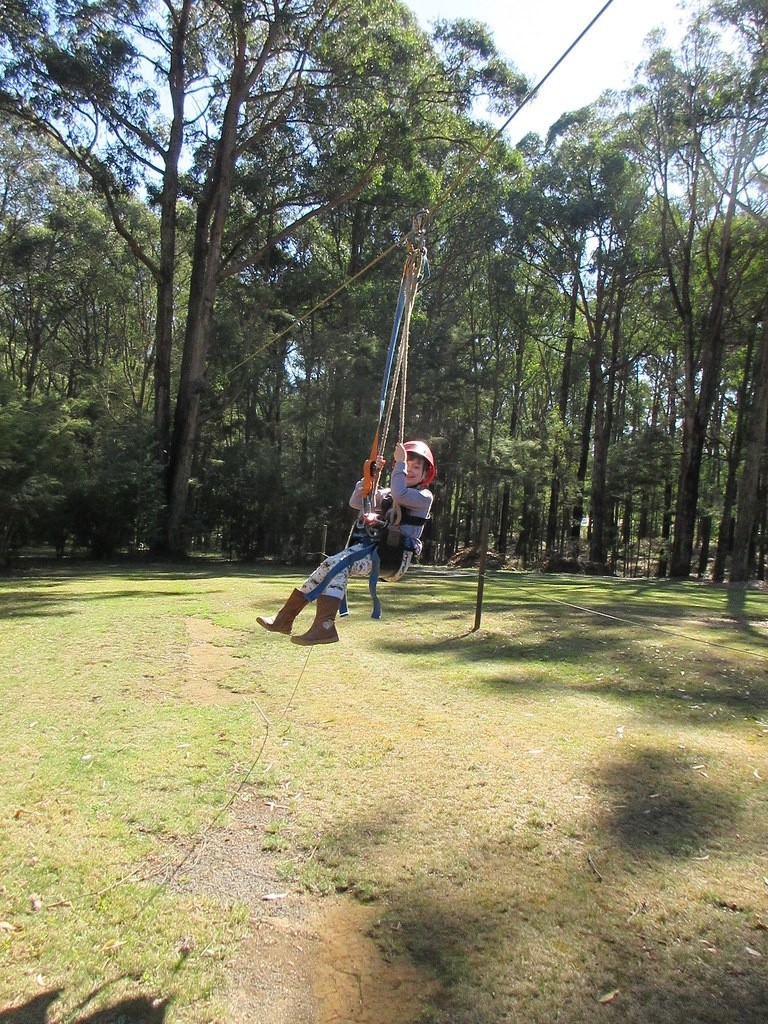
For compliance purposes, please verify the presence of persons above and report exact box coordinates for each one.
[256,441,435,645]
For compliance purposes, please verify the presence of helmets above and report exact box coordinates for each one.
[392,441,436,487]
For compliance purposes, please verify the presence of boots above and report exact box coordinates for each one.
[291,594,341,646]
[256,588,309,634]
